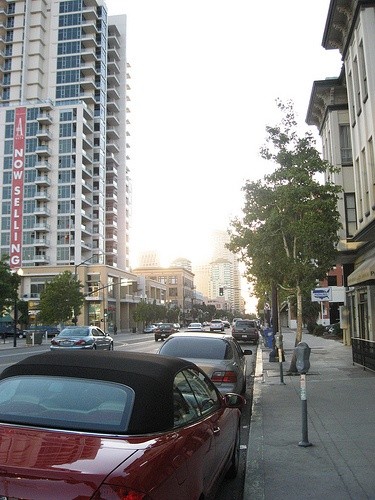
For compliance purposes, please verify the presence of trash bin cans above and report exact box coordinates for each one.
[258,329,267,346]
[26,333,43,344]
[263,327,275,348]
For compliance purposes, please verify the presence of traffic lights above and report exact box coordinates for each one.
[219,288,223,296]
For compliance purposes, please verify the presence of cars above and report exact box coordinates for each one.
[156,332,252,405]
[0,326,27,339]
[50,325,114,351]
[232,320,260,343]
[144,317,243,341]
[0,348,248,500]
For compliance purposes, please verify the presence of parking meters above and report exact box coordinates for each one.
[273,332,284,384]
[294,341,312,447]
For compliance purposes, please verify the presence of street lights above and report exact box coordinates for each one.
[10,268,24,346]
[74,253,137,326]
